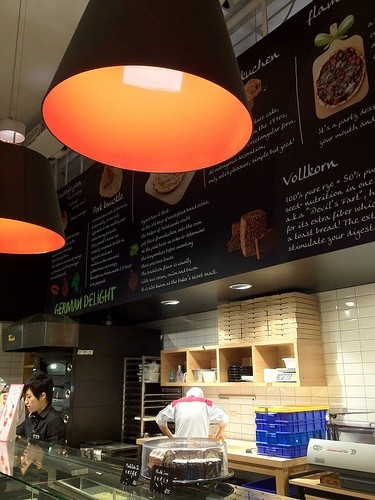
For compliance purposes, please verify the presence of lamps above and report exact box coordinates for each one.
[42,0,254,173]
[0,1,26,144]
[0,0,66,255]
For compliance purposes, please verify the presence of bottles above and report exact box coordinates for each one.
[176,365,184,382]
[169,371,174,382]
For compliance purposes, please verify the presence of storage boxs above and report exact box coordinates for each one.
[228,408,330,497]
[218,292,322,344]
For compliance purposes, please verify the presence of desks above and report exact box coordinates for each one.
[134,434,319,496]
[289,470,375,500]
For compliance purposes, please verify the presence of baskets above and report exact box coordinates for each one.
[253,404,330,458]
[242,478,293,497]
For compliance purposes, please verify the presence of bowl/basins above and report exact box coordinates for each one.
[281,357,296,368]
[227,365,252,382]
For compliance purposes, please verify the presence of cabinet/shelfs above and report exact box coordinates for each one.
[122,356,182,442]
[160,338,327,386]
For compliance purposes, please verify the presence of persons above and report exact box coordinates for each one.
[16,370,65,445]
[156,387,228,442]
[20,439,48,479]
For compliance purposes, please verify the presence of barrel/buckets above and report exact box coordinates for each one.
[263,368,278,383]
[192,369,212,383]
[203,371,215,383]
